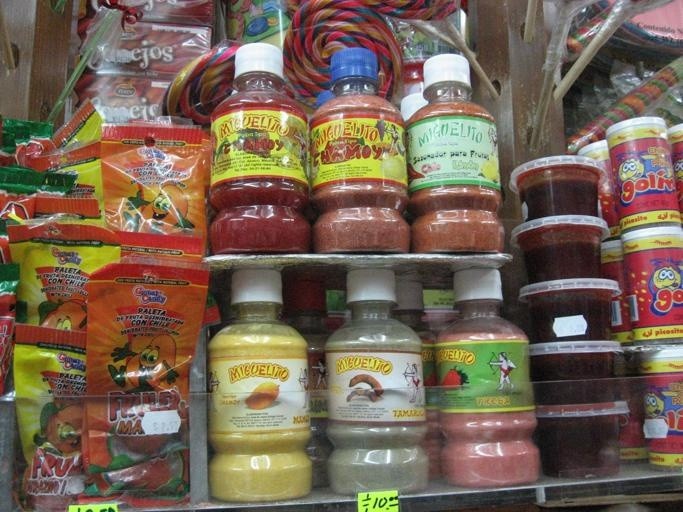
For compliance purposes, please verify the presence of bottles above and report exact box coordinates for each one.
[324,267,429,495]
[209,42,314,256]
[438,265,545,491]
[312,46,412,254]
[205,267,315,502]
[411,48,507,253]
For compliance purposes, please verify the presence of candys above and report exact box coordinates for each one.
[356,0,458,20]
[282,0,403,109]
[566,0,653,52]
[162,45,240,127]
[565,56,683,155]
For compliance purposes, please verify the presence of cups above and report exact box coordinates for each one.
[509,153,631,477]
